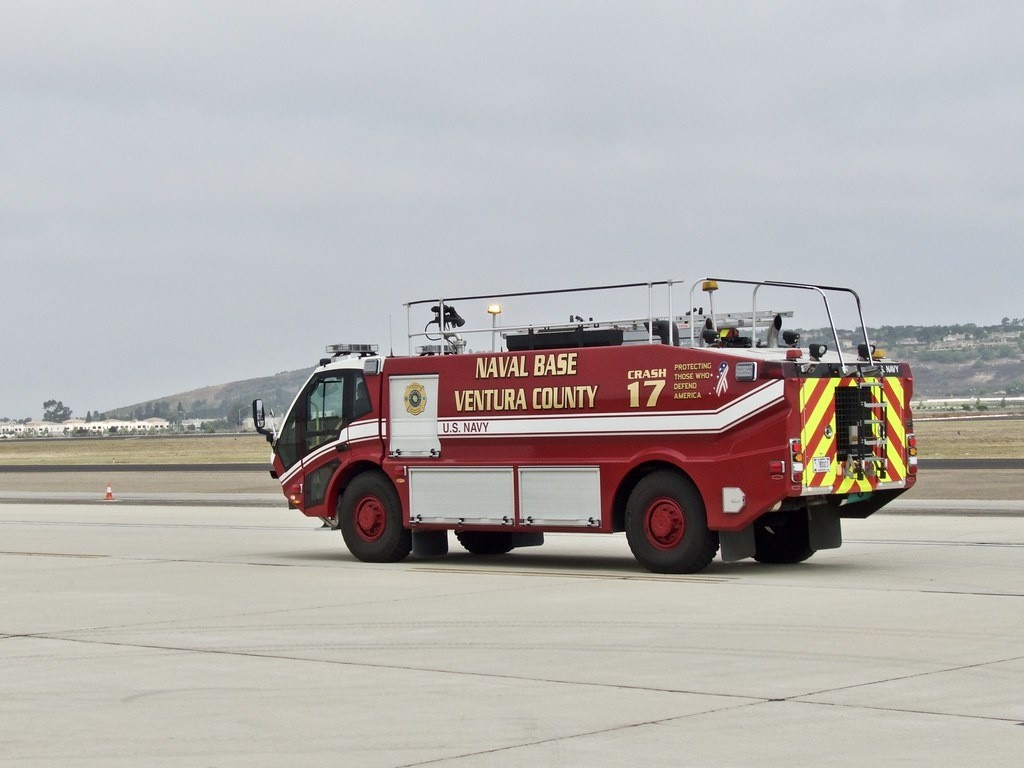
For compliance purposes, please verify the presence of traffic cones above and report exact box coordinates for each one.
[103,481,114,500]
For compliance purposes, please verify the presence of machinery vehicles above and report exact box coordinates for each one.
[251,277,918,575]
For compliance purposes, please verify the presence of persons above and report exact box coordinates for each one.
[355,381,371,416]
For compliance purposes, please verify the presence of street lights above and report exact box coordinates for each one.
[238,405,250,432]
